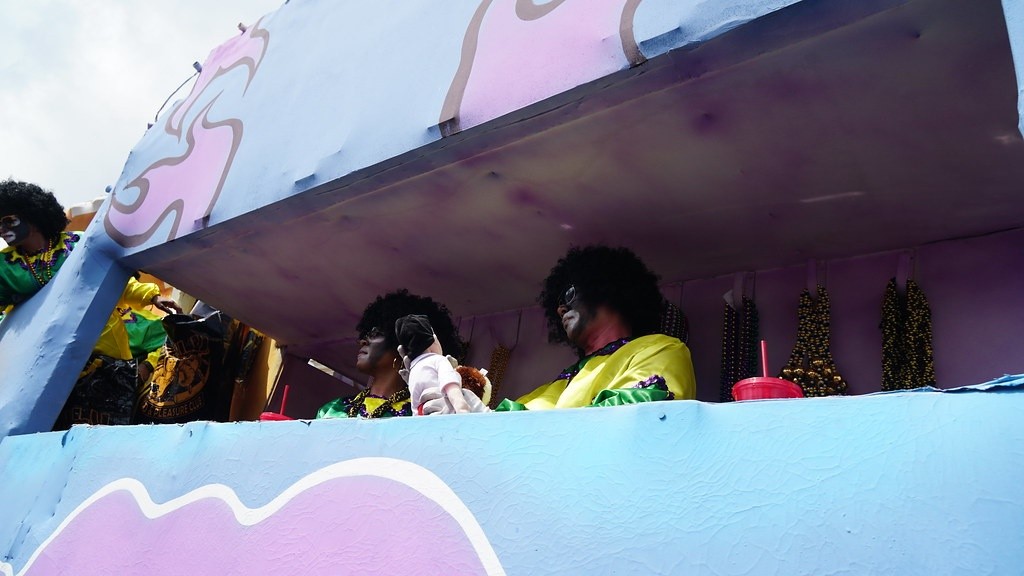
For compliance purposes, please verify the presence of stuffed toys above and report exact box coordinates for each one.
[394,312,473,415]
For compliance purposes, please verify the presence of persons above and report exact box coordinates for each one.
[398,240,698,411]
[0,178,187,429]
[310,290,466,415]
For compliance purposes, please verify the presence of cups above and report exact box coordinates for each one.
[732,377,803,401]
[260,412,293,421]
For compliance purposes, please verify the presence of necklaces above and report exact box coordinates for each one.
[655,302,691,346]
[482,343,511,410]
[347,385,409,418]
[24,238,53,286]
[721,293,760,400]
[780,283,848,398]
[878,276,938,389]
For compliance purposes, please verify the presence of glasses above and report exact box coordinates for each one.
[555,282,576,312]
[356,327,385,344]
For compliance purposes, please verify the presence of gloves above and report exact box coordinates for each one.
[419,383,494,415]
[396,344,457,385]
